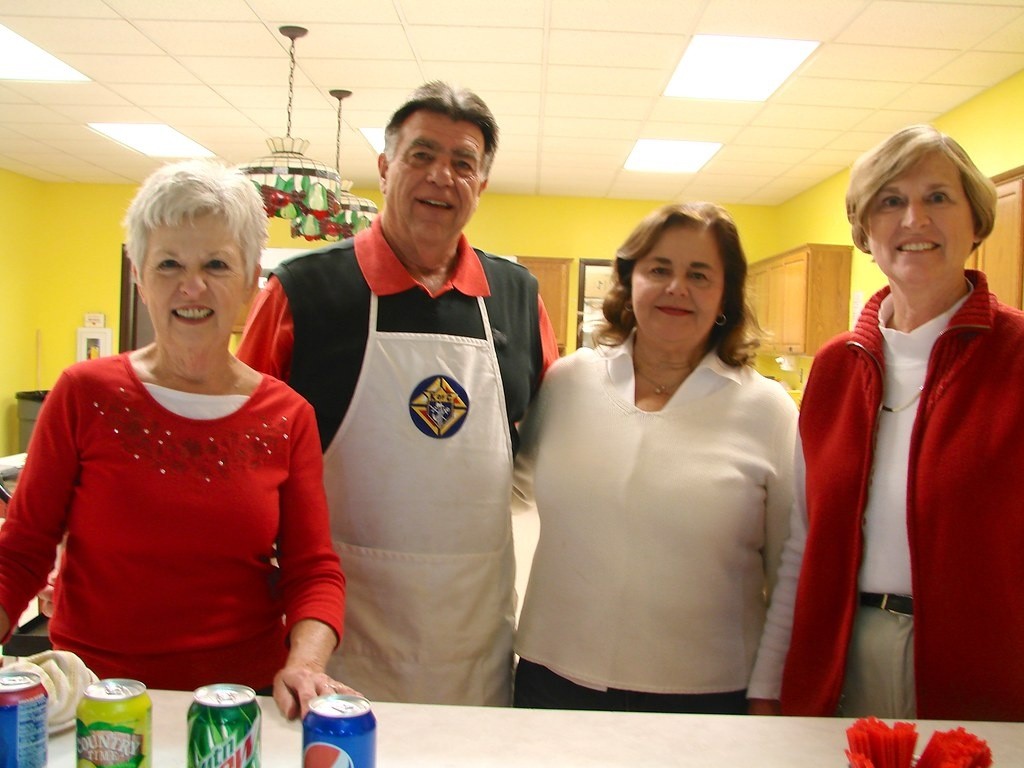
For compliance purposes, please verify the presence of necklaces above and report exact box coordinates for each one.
[631,361,691,399]
[873,385,924,417]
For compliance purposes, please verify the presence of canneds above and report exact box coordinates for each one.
[1,670,376,768]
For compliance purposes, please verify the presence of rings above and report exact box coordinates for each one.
[336,682,348,692]
[321,681,336,692]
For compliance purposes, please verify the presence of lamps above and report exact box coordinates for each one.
[291,90,377,243]
[240,26,341,218]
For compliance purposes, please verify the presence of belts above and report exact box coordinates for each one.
[856,591,914,618]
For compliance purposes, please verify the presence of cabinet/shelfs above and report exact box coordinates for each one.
[742,244,854,408]
[961,165,1024,311]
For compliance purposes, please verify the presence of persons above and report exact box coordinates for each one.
[741,121,1024,726]
[34,65,564,707]
[0,132,376,720]
[508,202,806,716]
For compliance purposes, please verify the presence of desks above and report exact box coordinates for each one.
[46,688,1024,768]
